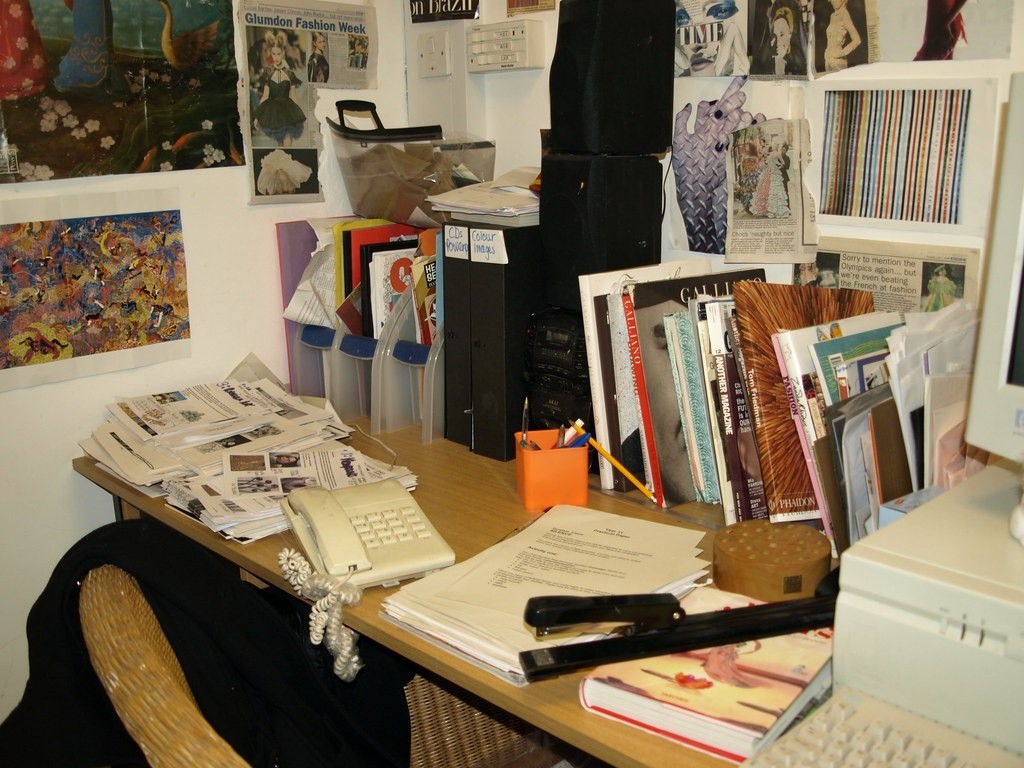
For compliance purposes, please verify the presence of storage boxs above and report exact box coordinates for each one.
[712,519,831,601]
[514,428,589,513]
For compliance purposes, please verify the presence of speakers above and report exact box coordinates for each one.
[538,0,678,316]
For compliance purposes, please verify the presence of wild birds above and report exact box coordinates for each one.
[158,0,223,72]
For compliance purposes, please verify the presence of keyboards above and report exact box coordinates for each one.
[740,690,1023,768]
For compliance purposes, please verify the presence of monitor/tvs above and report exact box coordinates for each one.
[963,73,1024,548]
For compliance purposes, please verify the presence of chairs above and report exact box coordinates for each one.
[78,565,565,768]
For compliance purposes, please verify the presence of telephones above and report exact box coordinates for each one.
[279,478,456,597]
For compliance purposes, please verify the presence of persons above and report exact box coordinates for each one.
[825,0,862,72]
[271,452,299,467]
[737,137,795,220]
[761,8,807,75]
[247,26,369,149]
[913,0,967,61]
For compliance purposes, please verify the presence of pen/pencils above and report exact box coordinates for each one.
[568,432,591,448]
[521,396,529,444]
[567,417,658,505]
[520,418,585,450]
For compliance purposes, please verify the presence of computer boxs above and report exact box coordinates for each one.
[833,457,1022,753]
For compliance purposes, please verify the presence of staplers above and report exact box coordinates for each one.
[518,592,837,685]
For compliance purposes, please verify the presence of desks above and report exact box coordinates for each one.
[72,414,841,768]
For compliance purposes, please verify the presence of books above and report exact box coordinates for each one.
[104,261,980,762]
